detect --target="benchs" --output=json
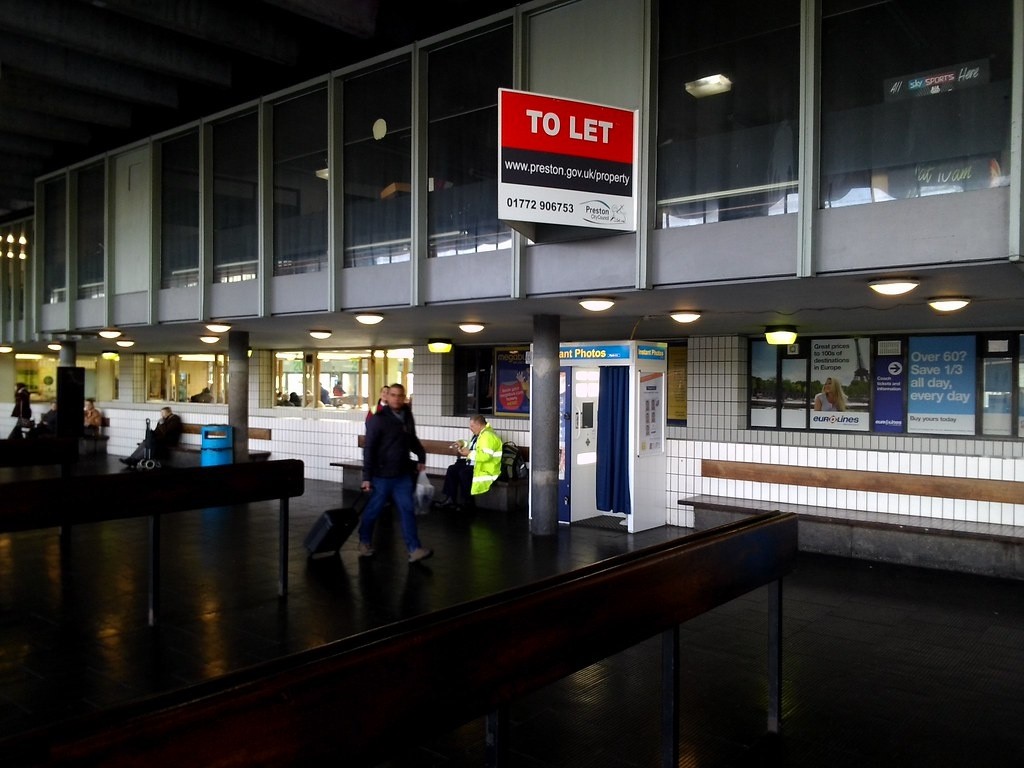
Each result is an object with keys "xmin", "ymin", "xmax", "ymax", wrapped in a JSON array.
[
  {"xmin": 138, "ymin": 423, "xmax": 272, "ymax": 469},
  {"xmin": 40, "ymin": 413, "xmax": 109, "ymax": 456},
  {"xmin": 676, "ymin": 459, "xmax": 1024, "ymax": 582},
  {"xmin": 330, "ymin": 436, "xmax": 528, "ymax": 513}
]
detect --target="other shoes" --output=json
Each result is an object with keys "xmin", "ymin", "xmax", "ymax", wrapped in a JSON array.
[
  {"xmin": 119, "ymin": 457, "xmax": 140, "ymax": 468},
  {"xmin": 437, "ymin": 497, "xmax": 456, "ymax": 507},
  {"xmin": 406, "ymin": 547, "xmax": 434, "ymax": 563},
  {"xmin": 356, "ymin": 540, "xmax": 376, "ymax": 557}
]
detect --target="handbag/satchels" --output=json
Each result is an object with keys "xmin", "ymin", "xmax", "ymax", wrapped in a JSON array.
[{"xmin": 416, "ymin": 469, "xmax": 434, "ymax": 508}]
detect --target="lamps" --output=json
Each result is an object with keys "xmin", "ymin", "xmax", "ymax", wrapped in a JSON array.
[
  {"xmin": 116, "ymin": 340, "xmax": 135, "ymax": 348},
  {"xmin": 310, "ymin": 330, "xmax": 332, "ymax": 339},
  {"xmin": 354, "ymin": 313, "xmax": 384, "ymax": 325},
  {"xmin": 669, "ymin": 311, "xmax": 702, "ymax": 324},
  {"xmin": 577, "ymin": 297, "xmax": 616, "ymax": 311},
  {"xmin": 764, "ymin": 325, "xmax": 799, "ymax": 344},
  {"xmin": 199, "ymin": 336, "xmax": 220, "ymax": 344},
  {"xmin": 684, "ymin": 72, "xmax": 732, "ymax": 100},
  {"xmin": 457, "ymin": 322, "xmax": 486, "ymax": 333},
  {"xmin": 102, "ymin": 350, "xmax": 118, "ymax": 360},
  {"xmin": 47, "ymin": 343, "xmax": 61, "ymax": 350},
  {"xmin": 926, "ymin": 298, "xmax": 971, "ymax": 312},
  {"xmin": 380, "ymin": 182, "xmax": 411, "ymax": 201},
  {"xmin": 867, "ymin": 279, "xmax": 920, "ymax": 295},
  {"xmin": 205, "ymin": 323, "xmax": 232, "ymax": 333},
  {"xmin": 98, "ymin": 330, "xmax": 122, "ymax": 339},
  {"xmin": 314, "ymin": 167, "xmax": 328, "ymax": 181},
  {"xmin": 0, "ymin": 346, "xmax": 13, "ymax": 354},
  {"xmin": 427, "ymin": 338, "xmax": 453, "ymax": 353}
]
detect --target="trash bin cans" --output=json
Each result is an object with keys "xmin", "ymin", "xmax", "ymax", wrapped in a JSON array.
[{"xmin": 200, "ymin": 425, "xmax": 233, "ymax": 466}]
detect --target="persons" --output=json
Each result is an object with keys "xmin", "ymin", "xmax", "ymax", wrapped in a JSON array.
[
  {"xmin": 289, "ymin": 392, "xmax": 301, "ymax": 407},
  {"xmin": 333, "ymin": 381, "xmax": 346, "ymax": 396},
  {"xmin": 305, "ymin": 389, "xmax": 313, "ymax": 405},
  {"xmin": 814, "ymin": 376, "xmax": 849, "ymax": 412},
  {"xmin": 179, "ymin": 379, "xmax": 187, "ymax": 402},
  {"xmin": 275, "ymin": 389, "xmax": 295, "ymax": 406},
  {"xmin": 365, "ymin": 385, "xmax": 390, "ymax": 427},
  {"xmin": 37, "ymin": 399, "xmax": 57, "ymax": 428},
  {"xmin": 118, "ymin": 407, "xmax": 183, "ymax": 467},
  {"xmin": 438, "ymin": 414, "xmax": 503, "ymax": 509},
  {"xmin": 333, "ymin": 398, "xmax": 345, "ymax": 409},
  {"xmin": 11, "ymin": 381, "xmax": 32, "ymax": 425},
  {"xmin": 356, "ymin": 383, "xmax": 434, "ymax": 562},
  {"xmin": 82, "ymin": 398, "xmax": 102, "ymax": 453},
  {"xmin": 320, "ymin": 382, "xmax": 330, "ymax": 404}
]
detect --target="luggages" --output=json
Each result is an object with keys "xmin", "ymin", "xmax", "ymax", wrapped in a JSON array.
[{"xmin": 303, "ymin": 484, "xmax": 374, "ymax": 557}]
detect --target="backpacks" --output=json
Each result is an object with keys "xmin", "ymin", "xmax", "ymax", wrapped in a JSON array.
[{"xmin": 499, "ymin": 441, "xmax": 529, "ymax": 482}]
